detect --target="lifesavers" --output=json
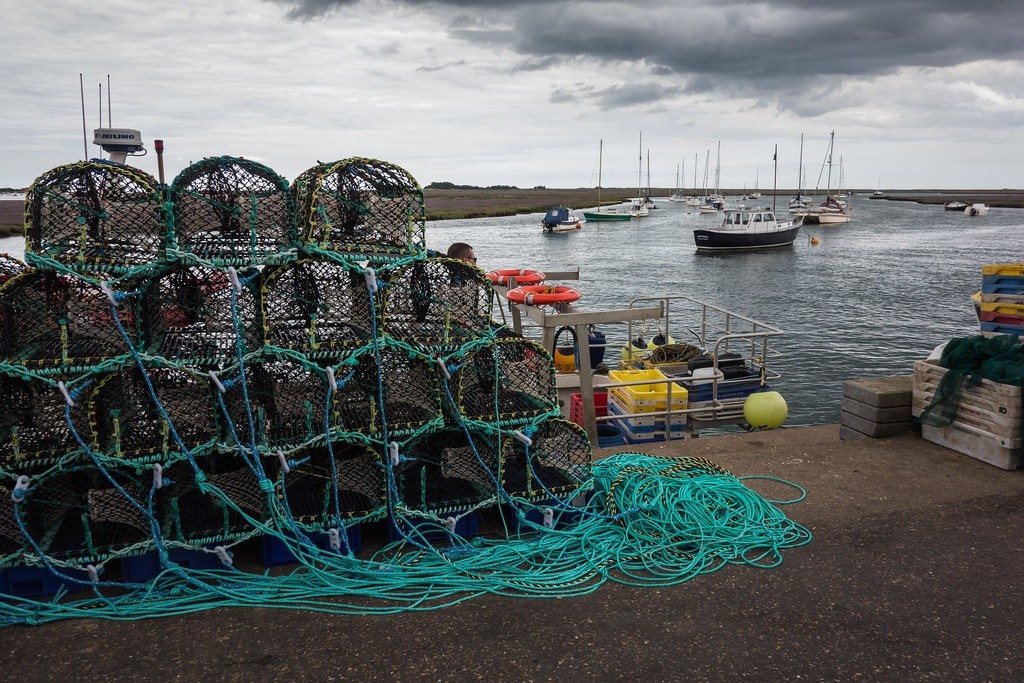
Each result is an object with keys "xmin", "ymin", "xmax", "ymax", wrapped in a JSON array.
[
  {"xmin": 506, "ymin": 285, "xmax": 581, "ymax": 305},
  {"xmin": 484, "ymin": 268, "xmax": 546, "ymax": 286}
]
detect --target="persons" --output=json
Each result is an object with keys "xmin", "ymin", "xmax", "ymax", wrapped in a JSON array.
[{"xmin": 447, "ymin": 242, "xmax": 477, "ymax": 268}]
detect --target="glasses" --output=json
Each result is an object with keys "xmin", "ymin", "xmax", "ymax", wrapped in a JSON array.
[{"xmin": 465, "ymin": 257, "xmax": 477, "ymax": 262}]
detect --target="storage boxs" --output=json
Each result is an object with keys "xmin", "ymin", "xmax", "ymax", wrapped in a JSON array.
[
  {"xmin": 912, "ymin": 262, "xmax": 1024, "ymax": 472},
  {"xmin": 569, "ymin": 351, "xmax": 766, "ymax": 445}
]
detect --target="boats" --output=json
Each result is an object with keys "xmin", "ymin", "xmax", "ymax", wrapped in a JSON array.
[
  {"xmin": 944, "ymin": 200, "xmax": 969, "ymax": 211},
  {"xmin": 874, "ymin": 190, "xmax": 882, "ymax": 195},
  {"xmin": 541, "ymin": 207, "xmax": 581, "ymax": 234},
  {"xmin": 693, "ymin": 207, "xmax": 804, "ymax": 249}
]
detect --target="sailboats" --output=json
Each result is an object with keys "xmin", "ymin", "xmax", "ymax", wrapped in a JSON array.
[
  {"xmin": 794, "ymin": 129, "xmax": 852, "ymax": 223},
  {"xmin": 669, "ymin": 140, "xmax": 725, "ymax": 213},
  {"xmin": 584, "ymin": 139, "xmax": 631, "ymax": 221},
  {"xmin": 788, "ymin": 130, "xmax": 813, "ymax": 212},
  {"xmin": 624, "ymin": 131, "xmax": 655, "ymax": 217},
  {"xmin": 742, "ymin": 182, "xmax": 747, "ymax": 200},
  {"xmin": 749, "ymin": 165, "xmax": 762, "ymax": 199}
]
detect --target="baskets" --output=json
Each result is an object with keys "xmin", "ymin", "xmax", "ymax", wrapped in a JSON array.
[
  {"xmin": 570, "ymin": 349, "xmax": 763, "ymax": 444},
  {"xmin": 971, "ymin": 263, "xmax": 1024, "ymax": 343}
]
